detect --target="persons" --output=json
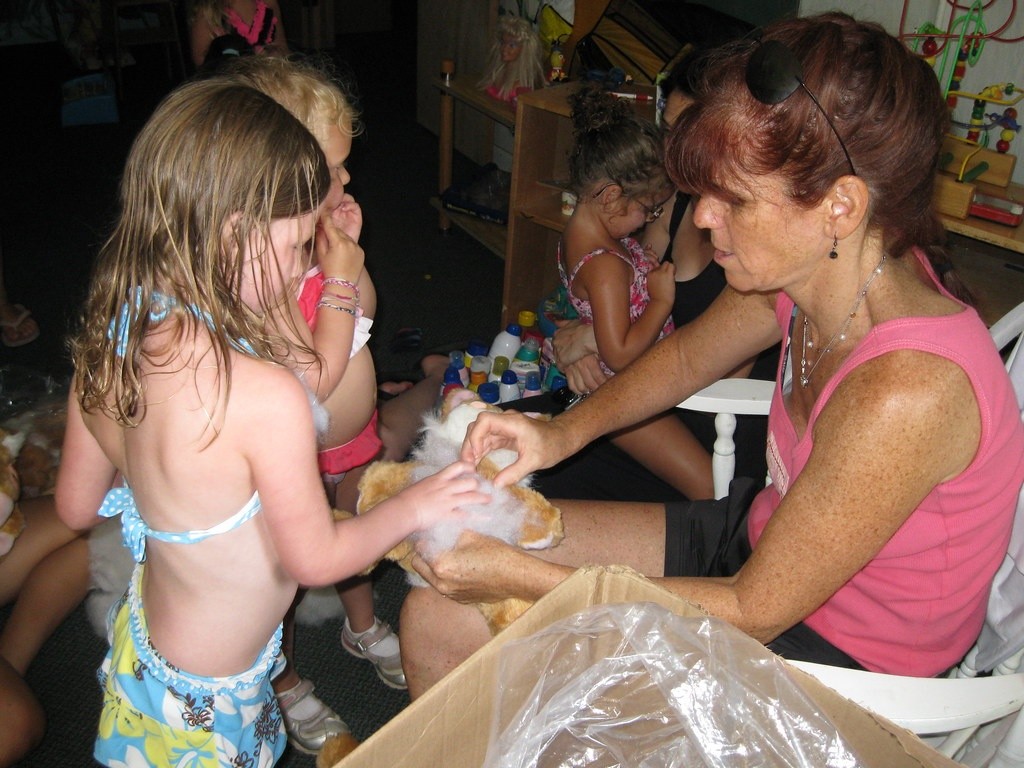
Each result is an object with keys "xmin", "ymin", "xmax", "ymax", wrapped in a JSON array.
[
  {"xmin": 397, "ymin": 10, "xmax": 1024, "ymax": 708},
  {"xmin": 0, "ymin": 0, "xmax": 413, "ymax": 767},
  {"xmin": 49, "ymin": 78, "xmax": 494, "ymax": 768}
]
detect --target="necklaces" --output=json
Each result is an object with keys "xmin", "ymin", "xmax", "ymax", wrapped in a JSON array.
[{"xmin": 799, "ymin": 256, "xmax": 886, "ymax": 389}]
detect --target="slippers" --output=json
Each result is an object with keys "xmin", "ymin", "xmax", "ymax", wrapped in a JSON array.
[{"xmin": 0, "ymin": 304, "xmax": 39, "ymax": 347}]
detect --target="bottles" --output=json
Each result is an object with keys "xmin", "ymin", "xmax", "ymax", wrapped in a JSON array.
[
  {"xmin": 432, "ymin": 35, "xmax": 468, "ymax": 81},
  {"xmin": 561, "ymin": 189, "xmax": 578, "ymax": 216},
  {"xmin": 436, "ymin": 309, "xmax": 581, "ymax": 418}
]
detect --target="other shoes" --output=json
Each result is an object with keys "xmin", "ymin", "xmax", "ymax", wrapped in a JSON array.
[
  {"xmin": 341, "ymin": 620, "xmax": 408, "ymax": 688},
  {"xmin": 276, "ymin": 678, "xmax": 350, "ymax": 756}
]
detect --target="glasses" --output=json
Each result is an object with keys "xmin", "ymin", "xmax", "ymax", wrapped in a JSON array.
[
  {"xmin": 593, "ymin": 182, "xmax": 664, "ymax": 220},
  {"xmin": 737, "ymin": 25, "xmax": 857, "ymax": 175}
]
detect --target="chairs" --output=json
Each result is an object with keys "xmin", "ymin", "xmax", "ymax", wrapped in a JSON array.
[{"xmin": 560, "ymin": 303, "xmax": 1024, "ymax": 768}]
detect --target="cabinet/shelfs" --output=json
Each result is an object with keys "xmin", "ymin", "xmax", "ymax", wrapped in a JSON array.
[
  {"xmin": 498, "ymin": 81, "xmax": 655, "ymax": 336},
  {"xmin": 426, "ymin": 60, "xmax": 517, "ymax": 260}
]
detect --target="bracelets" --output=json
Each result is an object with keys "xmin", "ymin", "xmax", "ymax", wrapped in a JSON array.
[
  {"xmin": 320, "ymin": 294, "xmax": 358, "ymax": 309},
  {"xmin": 317, "ymin": 302, "xmax": 355, "ymax": 317},
  {"xmin": 321, "ymin": 278, "xmax": 360, "ymax": 298}
]
